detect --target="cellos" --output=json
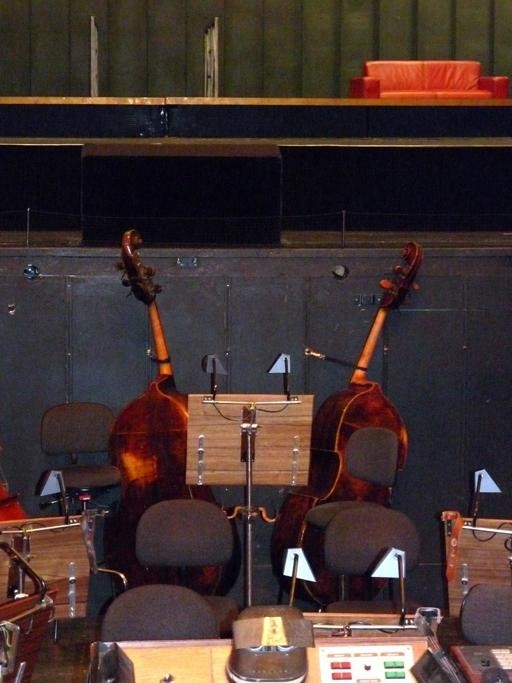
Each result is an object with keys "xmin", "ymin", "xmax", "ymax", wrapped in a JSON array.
[
  {"xmin": 271, "ymin": 240, "xmax": 423, "ymax": 606},
  {"xmin": 100, "ymin": 227, "xmax": 239, "ymax": 593}
]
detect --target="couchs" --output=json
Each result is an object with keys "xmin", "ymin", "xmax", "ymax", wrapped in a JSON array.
[{"xmin": 350, "ymin": 59, "xmax": 508, "ymax": 101}]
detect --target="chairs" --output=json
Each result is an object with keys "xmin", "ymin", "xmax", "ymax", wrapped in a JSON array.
[
  {"xmin": 129, "ymin": 496, "xmax": 240, "ymax": 639},
  {"xmin": 87, "ymin": 584, "xmax": 222, "ymax": 681},
  {"xmin": 323, "ymin": 503, "xmax": 424, "ymax": 616},
  {"xmin": 293, "ymin": 426, "xmax": 401, "ymax": 614},
  {"xmin": 39, "ymin": 401, "xmax": 129, "ymax": 645},
  {"xmin": 457, "ymin": 581, "xmax": 511, "ymax": 646}
]
